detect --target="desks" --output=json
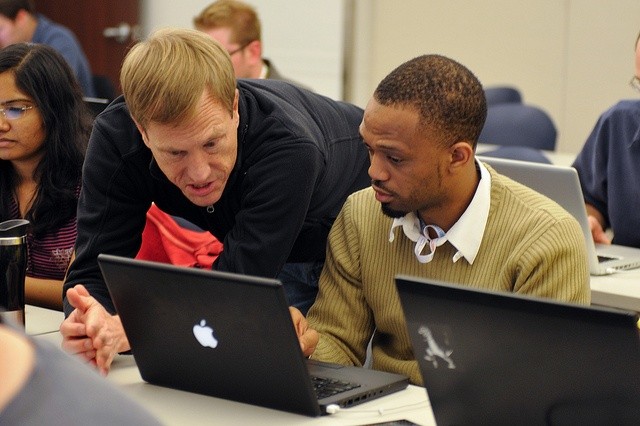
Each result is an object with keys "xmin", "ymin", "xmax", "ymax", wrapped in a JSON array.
[
  {"xmin": 591, "ymin": 242, "xmax": 640, "ymax": 315},
  {"xmin": 36, "ymin": 330, "xmax": 436, "ymax": 426},
  {"xmin": 24, "ymin": 303, "xmax": 71, "ymax": 334}
]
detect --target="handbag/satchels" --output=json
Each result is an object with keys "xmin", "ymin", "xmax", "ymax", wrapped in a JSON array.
[{"xmin": 135, "ymin": 202, "xmax": 224, "ymax": 270}]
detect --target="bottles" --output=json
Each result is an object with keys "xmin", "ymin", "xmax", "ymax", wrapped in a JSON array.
[{"xmin": 1, "ymin": 219, "xmax": 31, "ymax": 329}]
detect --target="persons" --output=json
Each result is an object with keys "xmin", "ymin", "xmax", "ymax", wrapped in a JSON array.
[
  {"xmin": 60, "ymin": 24, "xmax": 374, "ymax": 388},
  {"xmin": 193, "ymin": 0, "xmax": 302, "ymax": 84},
  {"xmin": 0, "ymin": 0, "xmax": 94, "ymax": 101},
  {"xmin": 570, "ymin": 29, "xmax": 639, "ymax": 249},
  {"xmin": 0, "ymin": 41, "xmax": 95, "ymax": 310},
  {"xmin": 285, "ymin": 52, "xmax": 591, "ymax": 387}
]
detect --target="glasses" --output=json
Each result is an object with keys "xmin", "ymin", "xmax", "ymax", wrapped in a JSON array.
[
  {"xmin": 0, "ymin": 104, "xmax": 37, "ymax": 120},
  {"xmin": 630, "ymin": 76, "xmax": 639, "ymax": 94},
  {"xmin": 229, "ymin": 42, "xmax": 251, "ymax": 55}
]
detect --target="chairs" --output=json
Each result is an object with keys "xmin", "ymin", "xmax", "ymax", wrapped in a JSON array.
[
  {"xmin": 484, "ymin": 87, "xmax": 521, "ymax": 105},
  {"xmin": 477, "ymin": 104, "xmax": 556, "ymax": 150}
]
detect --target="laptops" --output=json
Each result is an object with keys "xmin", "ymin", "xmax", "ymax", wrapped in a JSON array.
[
  {"xmin": 81, "ymin": 91, "xmax": 110, "ymax": 115},
  {"xmin": 392, "ymin": 273, "xmax": 634, "ymax": 425},
  {"xmin": 96, "ymin": 251, "xmax": 411, "ymax": 420},
  {"xmin": 477, "ymin": 154, "xmax": 640, "ymax": 279}
]
